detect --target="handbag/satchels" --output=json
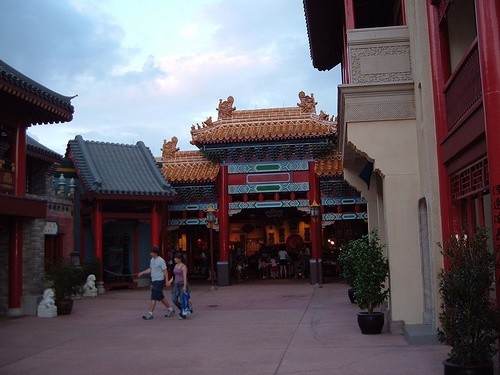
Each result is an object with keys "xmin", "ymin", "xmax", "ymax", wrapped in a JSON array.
[{"xmin": 180, "ymin": 291, "xmax": 193, "ymax": 316}]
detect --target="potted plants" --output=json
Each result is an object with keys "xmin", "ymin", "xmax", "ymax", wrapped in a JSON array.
[
  {"xmin": 434, "ymin": 221, "xmax": 500, "ymax": 375},
  {"xmin": 40, "ymin": 256, "xmax": 88, "ymax": 314},
  {"xmin": 337, "ymin": 225, "xmax": 390, "ymax": 334}
]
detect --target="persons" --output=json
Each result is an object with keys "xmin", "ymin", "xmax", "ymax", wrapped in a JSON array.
[
  {"xmin": 231, "ymin": 244, "xmax": 310, "ymax": 282},
  {"xmin": 167, "ymin": 253, "xmax": 189, "ymax": 320},
  {"xmin": 137, "ymin": 247, "xmax": 176, "ymax": 320}
]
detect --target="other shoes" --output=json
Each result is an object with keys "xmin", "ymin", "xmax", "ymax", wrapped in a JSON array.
[
  {"xmin": 177, "ymin": 314, "xmax": 185, "ymax": 320},
  {"xmin": 142, "ymin": 312, "xmax": 153, "ymax": 320},
  {"xmin": 164, "ymin": 309, "xmax": 175, "ymax": 318}
]
